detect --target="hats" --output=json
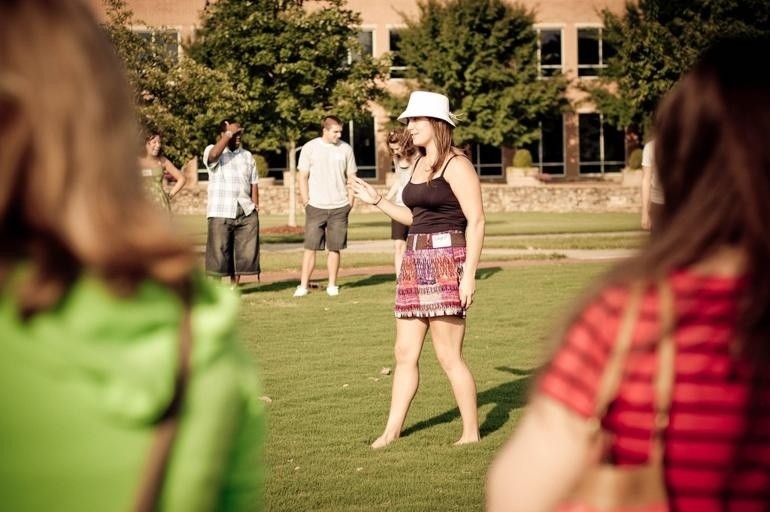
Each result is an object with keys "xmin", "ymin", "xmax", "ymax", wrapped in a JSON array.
[{"xmin": 403, "ymin": 91, "xmax": 455, "ymax": 124}]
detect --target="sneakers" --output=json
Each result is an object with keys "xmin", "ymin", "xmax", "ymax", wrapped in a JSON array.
[
  {"xmin": 326, "ymin": 285, "xmax": 339, "ymax": 297},
  {"xmin": 293, "ymin": 286, "xmax": 308, "ymax": 296}
]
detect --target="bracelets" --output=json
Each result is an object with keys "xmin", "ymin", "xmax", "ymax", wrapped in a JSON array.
[
  {"xmin": 374, "ymin": 196, "xmax": 384, "ymax": 205},
  {"xmin": 303, "ymin": 199, "xmax": 309, "ymax": 207}
]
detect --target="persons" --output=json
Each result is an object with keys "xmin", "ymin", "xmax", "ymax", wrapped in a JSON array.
[
  {"xmin": 483, "ymin": 36, "xmax": 770, "ymax": 511},
  {"xmin": 0, "ymin": 0, "xmax": 268, "ymax": 511},
  {"xmin": 348, "ymin": 90, "xmax": 485, "ymax": 449},
  {"xmin": 641, "ymin": 139, "xmax": 671, "ymax": 242},
  {"xmin": 202, "ymin": 119, "xmax": 261, "ymax": 289},
  {"xmin": 386, "ymin": 126, "xmax": 420, "ymax": 286},
  {"xmin": 294, "ymin": 115, "xmax": 358, "ymax": 297},
  {"xmin": 135, "ymin": 126, "xmax": 186, "ymax": 210}
]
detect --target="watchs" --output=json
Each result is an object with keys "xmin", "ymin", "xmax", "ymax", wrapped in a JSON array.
[
  {"xmin": 167, "ymin": 193, "xmax": 172, "ymax": 199},
  {"xmin": 225, "ymin": 129, "xmax": 233, "ymax": 138}
]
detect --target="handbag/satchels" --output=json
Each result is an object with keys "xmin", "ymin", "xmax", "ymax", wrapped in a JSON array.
[{"xmin": 548, "ymin": 266, "xmax": 673, "ymax": 512}]
